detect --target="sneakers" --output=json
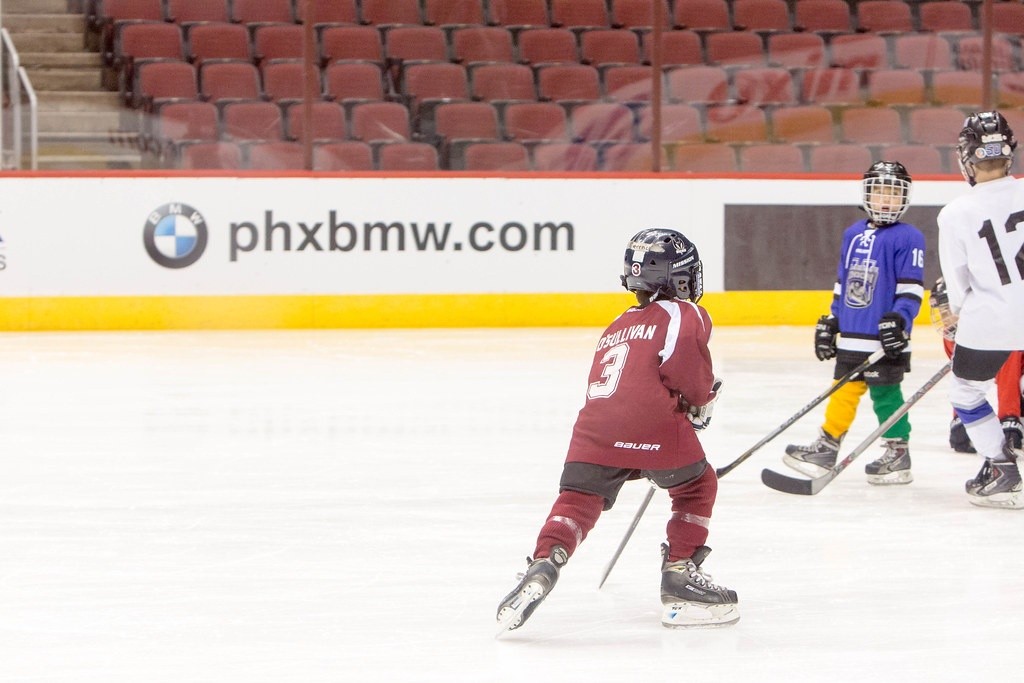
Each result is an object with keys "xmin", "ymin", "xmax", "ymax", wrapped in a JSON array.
[
  {"xmin": 783, "ymin": 427, "xmax": 848, "ymax": 480},
  {"xmin": 865, "ymin": 440, "xmax": 914, "ymax": 485},
  {"xmin": 493, "ymin": 544, "xmax": 567, "ymax": 639},
  {"xmin": 1001, "ymin": 416, "xmax": 1023, "ymax": 458},
  {"xmin": 965, "ymin": 457, "xmax": 1023, "ymax": 509},
  {"xmin": 949, "ymin": 415, "xmax": 977, "ymax": 454},
  {"xmin": 659, "ymin": 543, "xmax": 740, "ymax": 626}
]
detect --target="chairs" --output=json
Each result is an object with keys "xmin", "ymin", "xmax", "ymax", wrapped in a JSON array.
[{"xmin": 83, "ymin": 0, "xmax": 1024, "ymax": 172}]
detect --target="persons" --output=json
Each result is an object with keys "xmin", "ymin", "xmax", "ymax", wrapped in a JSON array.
[
  {"xmin": 497, "ymin": 229, "xmax": 740, "ymax": 632},
  {"xmin": 937, "ymin": 111, "xmax": 1024, "ymax": 507},
  {"xmin": 784, "ymin": 160, "xmax": 925, "ymax": 484},
  {"xmin": 929, "ymin": 279, "xmax": 1024, "ymax": 459}
]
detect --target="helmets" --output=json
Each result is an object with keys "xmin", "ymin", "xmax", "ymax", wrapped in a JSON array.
[
  {"xmin": 956, "ymin": 111, "xmax": 1018, "ymax": 187},
  {"xmin": 620, "ymin": 228, "xmax": 704, "ymax": 303},
  {"xmin": 930, "ymin": 277, "xmax": 959, "ymax": 342},
  {"xmin": 858, "ymin": 161, "xmax": 912, "ymax": 225}
]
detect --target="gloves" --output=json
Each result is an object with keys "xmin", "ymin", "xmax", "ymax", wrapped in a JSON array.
[
  {"xmin": 687, "ymin": 377, "xmax": 724, "ymax": 430},
  {"xmin": 814, "ymin": 315, "xmax": 839, "ymax": 361},
  {"xmin": 879, "ymin": 312, "xmax": 909, "ymax": 360}
]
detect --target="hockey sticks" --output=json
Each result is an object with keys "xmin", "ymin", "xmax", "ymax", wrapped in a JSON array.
[
  {"xmin": 597, "ymin": 404, "xmax": 700, "ymax": 590},
  {"xmin": 712, "ymin": 331, "xmax": 912, "ymax": 478},
  {"xmin": 761, "ymin": 358, "xmax": 955, "ymax": 496}
]
